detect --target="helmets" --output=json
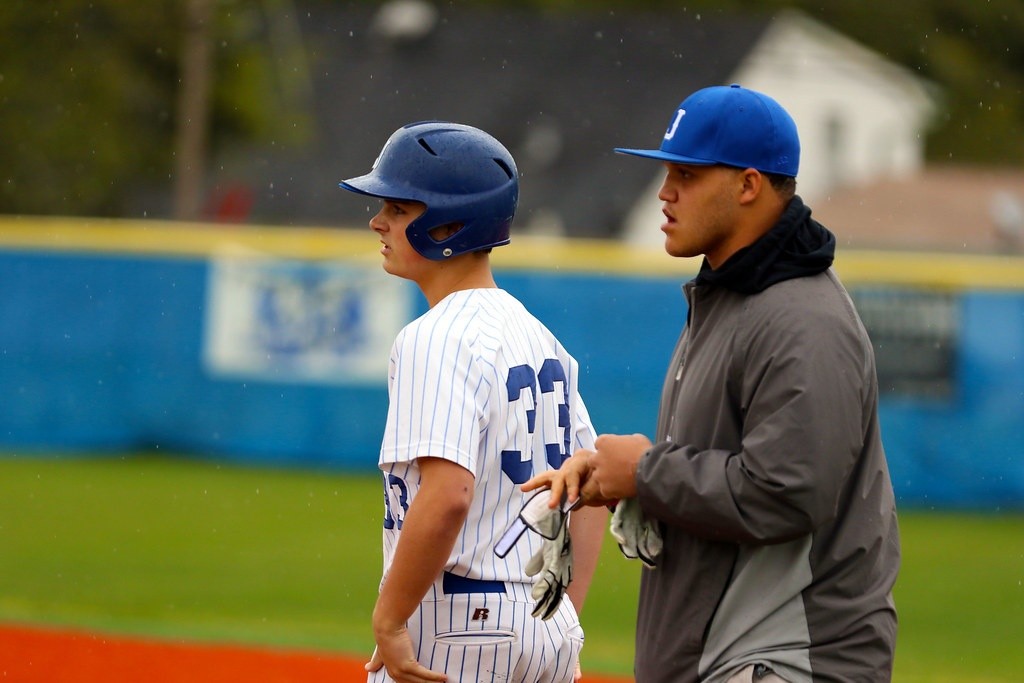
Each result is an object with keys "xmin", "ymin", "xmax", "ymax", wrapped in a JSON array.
[{"xmin": 339, "ymin": 119, "xmax": 518, "ymax": 259}]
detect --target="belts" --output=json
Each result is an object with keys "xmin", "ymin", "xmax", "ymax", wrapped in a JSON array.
[{"xmin": 444, "ymin": 571, "xmax": 506, "ymax": 594}]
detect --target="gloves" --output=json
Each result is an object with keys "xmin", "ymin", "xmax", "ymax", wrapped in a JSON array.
[
  {"xmin": 526, "ymin": 516, "xmax": 573, "ymax": 622},
  {"xmin": 610, "ymin": 497, "xmax": 664, "ymax": 568}
]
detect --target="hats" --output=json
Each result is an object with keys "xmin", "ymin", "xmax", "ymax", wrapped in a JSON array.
[{"xmin": 614, "ymin": 84, "xmax": 800, "ymax": 176}]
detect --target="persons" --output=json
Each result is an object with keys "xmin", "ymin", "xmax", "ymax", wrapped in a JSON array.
[
  {"xmin": 512, "ymin": 85, "xmax": 904, "ymax": 683},
  {"xmin": 334, "ymin": 120, "xmax": 615, "ymax": 683}
]
